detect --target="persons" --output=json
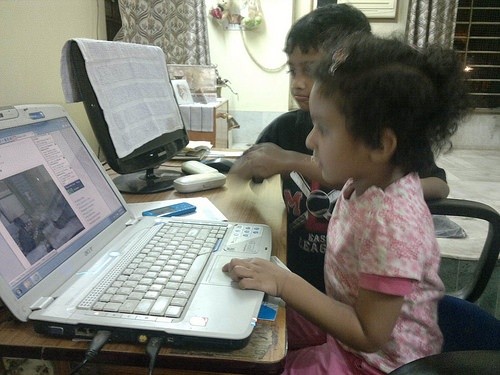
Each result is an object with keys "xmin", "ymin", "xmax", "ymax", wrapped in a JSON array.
[
  {"xmin": 226, "ymin": 4, "xmax": 450, "ymax": 290},
  {"xmin": 223, "ymin": 38, "xmax": 474, "ymax": 375}
]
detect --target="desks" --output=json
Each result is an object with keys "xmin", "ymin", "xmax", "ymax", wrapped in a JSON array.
[{"xmin": 0, "ymin": 148, "xmax": 287, "ymax": 375}]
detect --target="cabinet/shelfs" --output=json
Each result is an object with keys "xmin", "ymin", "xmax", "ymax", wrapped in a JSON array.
[{"xmin": 179, "ymin": 99, "xmax": 229, "ymax": 148}]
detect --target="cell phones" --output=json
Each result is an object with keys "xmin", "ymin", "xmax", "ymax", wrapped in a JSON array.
[{"xmin": 142, "ymin": 200, "xmax": 193, "ymax": 218}]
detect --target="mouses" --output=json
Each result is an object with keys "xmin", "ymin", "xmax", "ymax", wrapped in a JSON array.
[{"xmin": 200, "ymin": 157, "xmax": 235, "ymax": 171}]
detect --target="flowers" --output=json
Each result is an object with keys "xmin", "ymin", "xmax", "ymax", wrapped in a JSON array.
[{"xmin": 209, "ymin": 0, "xmax": 263, "ymax": 31}]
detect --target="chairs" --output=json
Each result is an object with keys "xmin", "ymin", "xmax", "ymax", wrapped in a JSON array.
[{"xmin": 387, "ymin": 198, "xmax": 500, "ymax": 375}]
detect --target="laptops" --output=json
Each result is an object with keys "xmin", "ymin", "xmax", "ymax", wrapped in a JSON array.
[{"xmin": 0, "ymin": 103, "xmax": 275, "ymax": 350}]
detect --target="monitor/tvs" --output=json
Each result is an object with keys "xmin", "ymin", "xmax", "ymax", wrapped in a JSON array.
[{"xmin": 69, "ymin": 41, "xmax": 189, "ymax": 194}]
